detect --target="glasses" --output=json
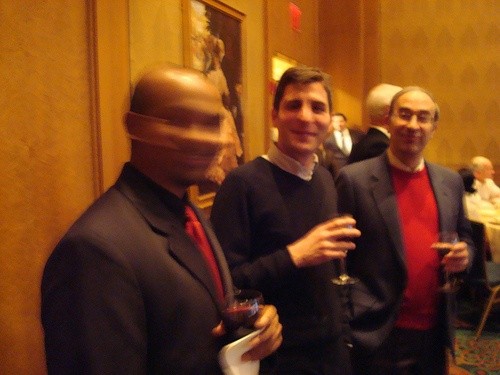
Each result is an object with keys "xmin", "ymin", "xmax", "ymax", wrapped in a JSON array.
[{"xmin": 391, "ymin": 111, "xmax": 436, "ymax": 123}]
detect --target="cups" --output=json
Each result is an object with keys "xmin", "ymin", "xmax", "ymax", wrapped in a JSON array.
[{"xmin": 218, "ymin": 289, "xmax": 264, "ymax": 342}]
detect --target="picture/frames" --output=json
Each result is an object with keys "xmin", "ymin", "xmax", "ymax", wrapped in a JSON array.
[{"xmin": 180, "ymin": 0, "xmax": 249, "ymax": 209}]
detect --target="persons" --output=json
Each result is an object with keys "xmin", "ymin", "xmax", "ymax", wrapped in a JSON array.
[
  {"xmin": 334, "ymin": 86, "xmax": 475, "ymax": 375},
  {"xmin": 210, "ymin": 65, "xmax": 361, "ymax": 375},
  {"xmin": 471, "ymin": 156, "xmax": 500, "ymax": 207},
  {"xmin": 457, "ymin": 168, "xmax": 492, "ymax": 209},
  {"xmin": 349, "ymin": 83, "xmax": 403, "ymax": 163},
  {"xmin": 324, "ymin": 113, "xmax": 365, "ymax": 170},
  {"xmin": 39, "ymin": 67, "xmax": 282, "ymax": 375}
]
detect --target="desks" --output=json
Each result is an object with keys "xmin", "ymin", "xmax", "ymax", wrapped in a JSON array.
[{"xmin": 466, "ymin": 196, "xmax": 500, "ymax": 263}]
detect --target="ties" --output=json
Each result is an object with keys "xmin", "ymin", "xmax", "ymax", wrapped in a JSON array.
[
  {"xmin": 341, "ymin": 131, "xmax": 348, "ymax": 157},
  {"xmin": 183, "ymin": 203, "xmax": 226, "ymax": 306}
]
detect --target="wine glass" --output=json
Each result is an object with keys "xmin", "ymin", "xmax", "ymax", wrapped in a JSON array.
[
  {"xmin": 326, "ymin": 214, "xmax": 358, "ymax": 285},
  {"xmin": 430, "ymin": 230, "xmax": 463, "ymax": 293}
]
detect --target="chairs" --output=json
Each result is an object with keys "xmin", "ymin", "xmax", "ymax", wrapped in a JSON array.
[{"xmin": 466, "ymin": 218, "xmax": 500, "ymax": 340}]
